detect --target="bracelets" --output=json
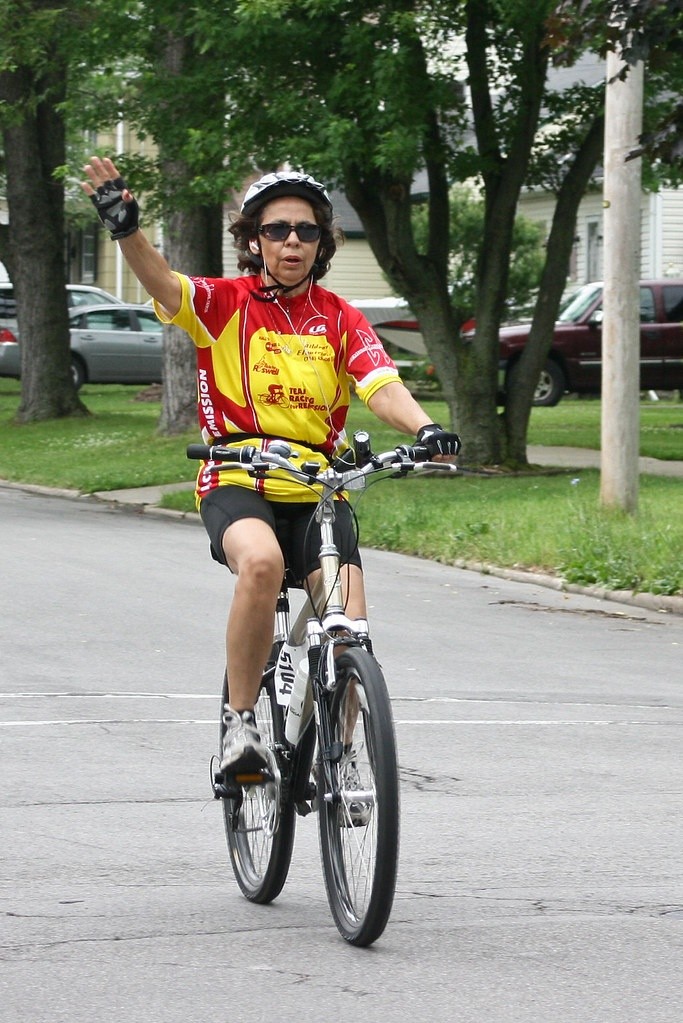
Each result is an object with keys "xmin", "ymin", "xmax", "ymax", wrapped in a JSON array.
[{"xmin": 110, "ymin": 225, "xmax": 138, "ymax": 241}]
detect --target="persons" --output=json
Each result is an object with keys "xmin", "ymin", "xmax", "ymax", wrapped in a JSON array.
[{"xmin": 76, "ymin": 152, "xmax": 463, "ymax": 830}]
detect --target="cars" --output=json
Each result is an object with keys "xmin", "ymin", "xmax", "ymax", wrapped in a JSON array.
[
  {"xmin": 0, "ymin": 303, "xmax": 172, "ymax": 392},
  {"xmin": 0, "ymin": 283, "xmax": 123, "ymax": 313}
]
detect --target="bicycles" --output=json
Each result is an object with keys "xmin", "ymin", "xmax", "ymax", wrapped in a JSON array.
[{"xmin": 183, "ymin": 430, "xmax": 463, "ymax": 949}]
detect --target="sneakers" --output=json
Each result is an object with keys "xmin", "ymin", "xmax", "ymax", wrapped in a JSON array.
[
  {"xmin": 311, "ymin": 756, "xmax": 372, "ymax": 828},
  {"xmin": 220, "ymin": 722, "xmax": 268, "ymax": 776}
]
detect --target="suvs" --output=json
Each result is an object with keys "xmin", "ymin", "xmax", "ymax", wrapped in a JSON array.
[{"xmin": 460, "ymin": 279, "xmax": 683, "ymax": 408}]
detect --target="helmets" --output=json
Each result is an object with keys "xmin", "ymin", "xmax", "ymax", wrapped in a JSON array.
[{"xmin": 239, "ymin": 172, "xmax": 334, "ymax": 225}]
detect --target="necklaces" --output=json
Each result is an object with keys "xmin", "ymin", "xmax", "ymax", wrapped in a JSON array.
[{"xmin": 259, "ymin": 271, "xmax": 313, "ymax": 354}]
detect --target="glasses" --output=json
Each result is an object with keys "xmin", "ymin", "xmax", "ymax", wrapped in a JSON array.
[{"xmin": 254, "ymin": 223, "xmax": 324, "ymax": 243}]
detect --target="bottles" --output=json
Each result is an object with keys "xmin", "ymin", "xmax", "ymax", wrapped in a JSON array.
[{"xmin": 285, "ymin": 657, "xmax": 309, "ymax": 746}]
